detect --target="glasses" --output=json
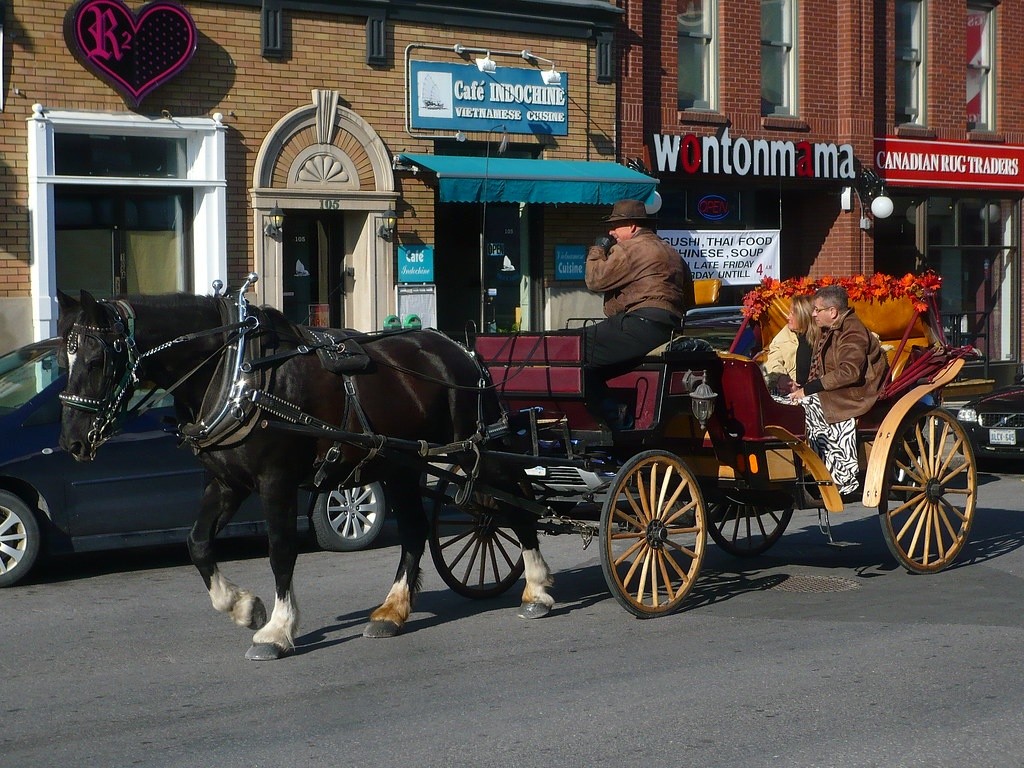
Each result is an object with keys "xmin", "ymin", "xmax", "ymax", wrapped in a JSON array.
[{"xmin": 813, "ymin": 306, "xmax": 835, "ymax": 314}]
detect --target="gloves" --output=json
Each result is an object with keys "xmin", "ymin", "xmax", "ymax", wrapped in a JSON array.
[{"xmin": 595, "ymin": 235, "xmax": 617, "ymax": 255}]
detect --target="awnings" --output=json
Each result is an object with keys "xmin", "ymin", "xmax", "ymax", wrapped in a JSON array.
[{"xmin": 400, "ymin": 154, "xmax": 658, "ymax": 205}]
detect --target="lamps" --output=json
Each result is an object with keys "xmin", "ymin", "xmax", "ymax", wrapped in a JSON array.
[
  {"xmin": 264, "ymin": 200, "xmax": 292, "ymax": 239},
  {"xmin": 859, "ymin": 168, "xmax": 893, "ymax": 219},
  {"xmin": 454, "ymin": 44, "xmax": 496, "ymax": 74},
  {"xmin": 520, "ymin": 49, "xmax": 561, "ymax": 86},
  {"xmin": 379, "ymin": 202, "xmax": 397, "ymax": 238},
  {"xmin": 644, "ymin": 190, "xmax": 662, "ymax": 214}
]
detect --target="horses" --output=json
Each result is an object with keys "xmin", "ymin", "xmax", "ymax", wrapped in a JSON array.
[{"xmin": 55, "ymin": 287, "xmax": 555, "ymax": 662}]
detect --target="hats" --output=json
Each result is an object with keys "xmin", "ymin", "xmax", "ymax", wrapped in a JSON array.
[{"xmin": 601, "ymin": 200, "xmax": 654, "ymax": 222}]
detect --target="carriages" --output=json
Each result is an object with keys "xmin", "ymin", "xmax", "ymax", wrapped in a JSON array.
[{"xmin": 58, "ymin": 271, "xmax": 996, "ymax": 662}]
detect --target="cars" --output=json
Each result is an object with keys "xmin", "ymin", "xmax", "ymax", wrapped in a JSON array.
[
  {"xmin": 521, "ymin": 455, "xmax": 722, "ymax": 527},
  {"xmin": 954, "ymin": 373, "xmax": 1024, "ymax": 471},
  {"xmin": 1, "ymin": 335, "xmax": 432, "ymax": 589}
]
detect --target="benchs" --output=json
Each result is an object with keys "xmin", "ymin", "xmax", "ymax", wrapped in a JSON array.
[
  {"xmin": 729, "ymin": 269, "xmax": 950, "ymax": 401},
  {"xmin": 593, "ymin": 334, "xmax": 723, "ymax": 428}
]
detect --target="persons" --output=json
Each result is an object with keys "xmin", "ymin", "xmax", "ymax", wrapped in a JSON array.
[
  {"xmin": 559, "ymin": 200, "xmax": 690, "ymax": 432},
  {"xmin": 763, "ymin": 286, "xmax": 889, "ymax": 501}
]
